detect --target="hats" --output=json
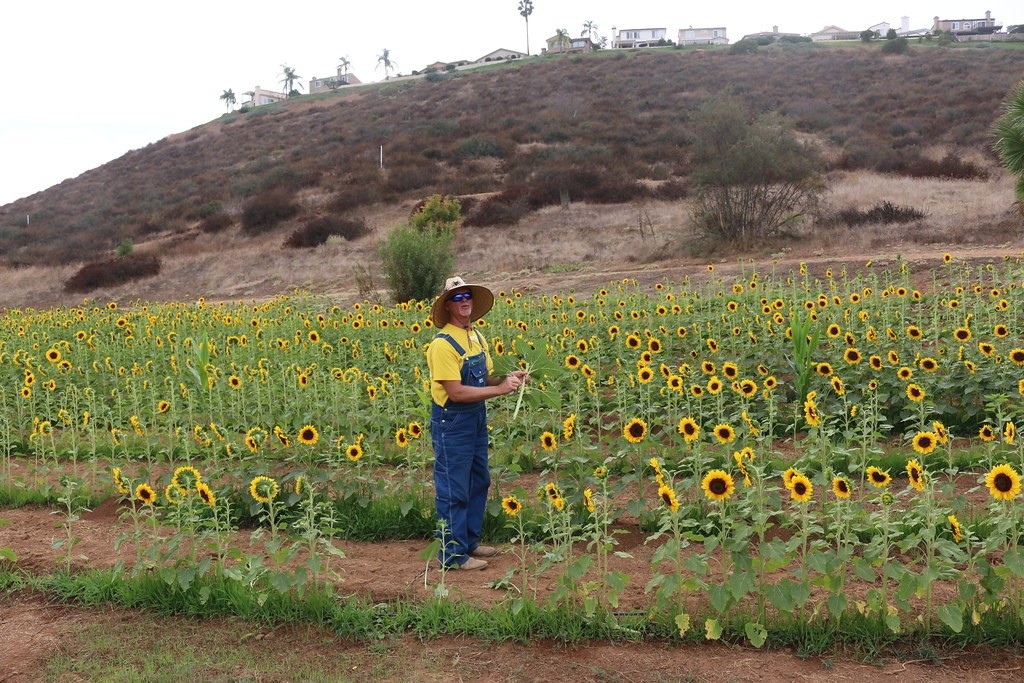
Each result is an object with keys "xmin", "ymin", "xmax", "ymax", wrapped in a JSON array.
[{"xmin": 431, "ymin": 277, "xmax": 496, "ymax": 329}]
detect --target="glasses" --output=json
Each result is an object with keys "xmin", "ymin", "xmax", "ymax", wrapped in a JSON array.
[{"xmin": 445, "ymin": 293, "xmax": 472, "ymax": 302}]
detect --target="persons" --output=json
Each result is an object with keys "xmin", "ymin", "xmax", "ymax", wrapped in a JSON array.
[{"xmin": 427, "ymin": 276, "xmax": 530, "ymax": 571}]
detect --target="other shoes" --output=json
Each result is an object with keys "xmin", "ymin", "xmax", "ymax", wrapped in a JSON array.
[
  {"xmin": 472, "ymin": 545, "xmax": 496, "ymax": 556},
  {"xmin": 441, "ymin": 557, "xmax": 489, "ymax": 571}
]
